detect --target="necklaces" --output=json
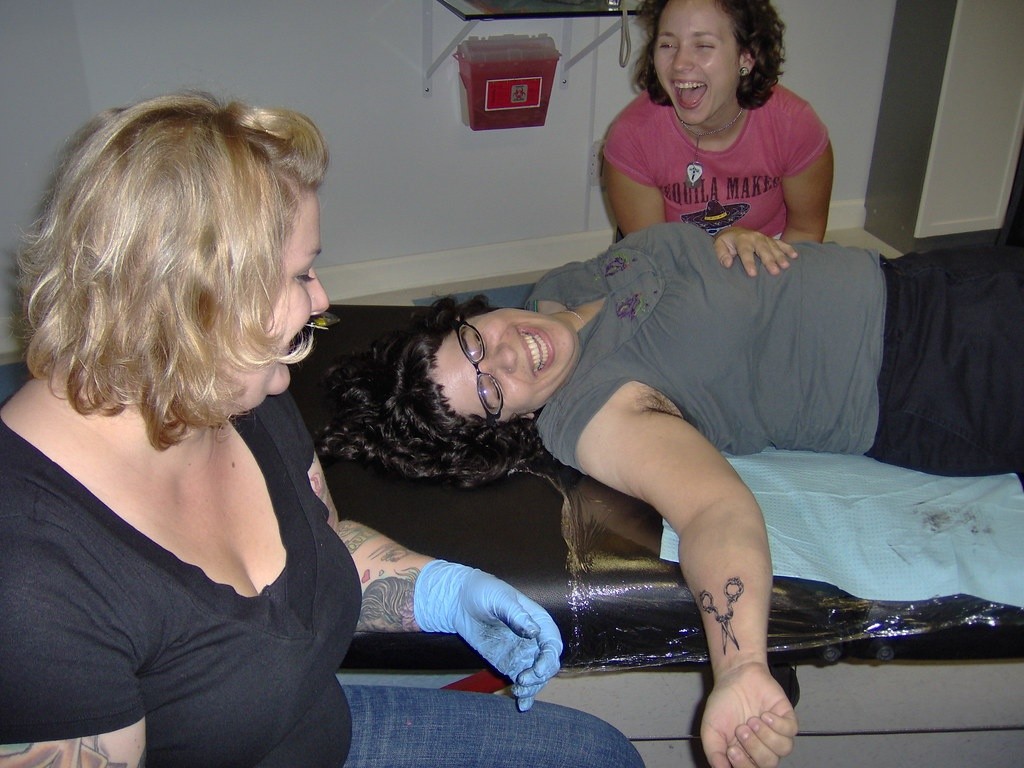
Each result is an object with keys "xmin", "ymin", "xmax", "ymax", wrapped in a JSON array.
[
  {"xmin": 681, "ymin": 109, "xmax": 744, "ymax": 189},
  {"xmin": 562, "ymin": 310, "xmax": 585, "ymax": 325}
]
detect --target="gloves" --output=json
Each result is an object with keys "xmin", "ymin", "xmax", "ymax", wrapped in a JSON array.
[{"xmin": 412, "ymin": 559, "xmax": 564, "ymax": 713}]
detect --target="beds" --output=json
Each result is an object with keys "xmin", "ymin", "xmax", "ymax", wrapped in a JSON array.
[{"xmin": 288, "ymin": 305, "xmax": 1024, "ymax": 767}]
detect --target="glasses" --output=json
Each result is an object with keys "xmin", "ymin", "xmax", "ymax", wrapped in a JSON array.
[{"xmin": 452, "ymin": 320, "xmax": 504, "ymax": 427}]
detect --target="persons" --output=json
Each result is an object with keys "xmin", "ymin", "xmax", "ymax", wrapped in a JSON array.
[
  {"xmin": 602, "ymin": 0, "xmax": 833, "ymax": 245},
  {"xmin": 320, "ymin": 221, "xmax": 1024, "ymax": 768},
  {"xmin": 0, "ymin": 94, "xmax": 647, "ymax": 768}
]
